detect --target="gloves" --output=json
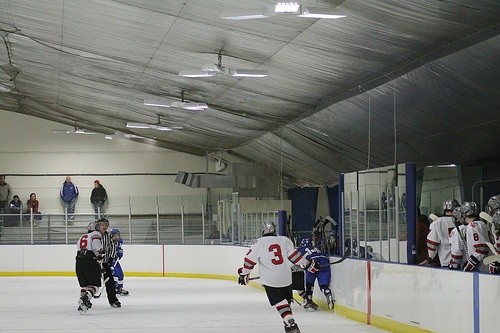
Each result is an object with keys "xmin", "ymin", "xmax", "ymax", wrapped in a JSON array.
[
  {"xmin": 118, "ymin": 249, "xmax": 123, "ymax": 259},
  {"xmin": 110, "ymin": 267, "xmax": 114, "ymax": 275},
  {"xmin": 424, "ymin": 256, "xmax": 499, "ymax": 274},
  {"xmin": 302, "ymin": 259, "xmax": 320, "ymax": 273},
  {"xmin": 237, "ymin": 268, "xmax": 250, "ymax": 285}
]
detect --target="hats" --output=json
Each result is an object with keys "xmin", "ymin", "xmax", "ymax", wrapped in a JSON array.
[{"xmin": 93, "ymin": 180, "xmax": 99, "ymax": 183}]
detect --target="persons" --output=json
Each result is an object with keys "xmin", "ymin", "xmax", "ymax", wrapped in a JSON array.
[
  {"xmin": 381, "ymin": 191, "xmax": 388, "ymax": 221},
  {"xmin": 298, "ymin": 231, "xmax": 335, "ymax": 309},
  {"xmin": 74, "ymin": 216, "xmax": 110, "ymax": 312},
  {"xmin": 60, "ymin": 176, "xmax": 79, "ymax": 224},
  {"xmin": 25, "ymin": 193, "xmax": 43, "ymax": 223},
  {"xmin": 8, "ymin": 194, "xmax": 26, "ymax": 224},
  {"xmin": 459, "ymin": 201, "xmax": 495, "ymax": 273},
  {"xmin": 447, "ymin": 207, "xmax": 473, "ymax": 271},
  {"xmin": 344, "ymin": 237, "xmax": 368, "ymax": 258},
  {"xmin": 239, "ymin": 220, "xmax": 320, "ymax": 333},
  {"xmin": 418, "ymin": 198, "xmax": 463, "ymax": 267},
  {"xmin": 322, "ymin": 233, "xmax": 341, "ymax": 255},
  {"xmin": 106, "ymin": 228, "xmax": 129, "ymax": 295},
  {"xmin": 0, "ymin": 174, "xmax": 12, "ymax": 225},
  {"xmin": 90, "ymin": 179, "xmax": 109, "ymax": 222},
  {"xmin": 85, "ymin": 221, "xmax": 121, "ymax": 308},
  {"xmin": 486, "ymin": 196, "xmax": 500, "ymax": 275}
]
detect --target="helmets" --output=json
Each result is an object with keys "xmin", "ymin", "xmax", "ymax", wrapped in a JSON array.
[
  {"xmin": 442, "ymin": 194, "xmax": 500, "ymax": 225},
  {"xmin": 87, "ymin": 218, "xmax": 121, "ymax": 241},
  {"xmin": 300, "ymin": 237, "xmax": 312, "ymax": 248},
  {"xmin": 364, "ymin": 246, "xmax": 373, "ymax": 251},
  {"xmin": 260, "ymin": 221, "xmax": 277, "ymax": 235},
  {"xmin": 344, "ymin": 237, "xmax": 358, "ymax": 247}
]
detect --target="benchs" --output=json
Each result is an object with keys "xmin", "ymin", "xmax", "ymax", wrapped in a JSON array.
[{"xmin": 1, "ymin": 211, "xmax": 230, "ymax": 245}]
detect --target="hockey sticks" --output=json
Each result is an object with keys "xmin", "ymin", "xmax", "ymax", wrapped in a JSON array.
[
  {"xmin": 93, "ymin": 256, "xmax": 120, "ymax": 299},
  {"xmin": 427, "ymin": 210, "xmax": 500, "ymax": 275},
  {"xmin": 248, "ymin": 249, "xmax": 350, "ymax": 281}
]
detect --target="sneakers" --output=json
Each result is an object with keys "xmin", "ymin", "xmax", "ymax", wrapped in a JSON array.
[
  {"xmin": 284, "ymin": 323, "xmax": 300, "ymax": 333},
  {"xmin": 77, "ymin": 288, "xmax": 129, "ymax": 314},
  {"xmin": 304, "ymin": 294, "xmax": 334, "ymax": 311}
]
